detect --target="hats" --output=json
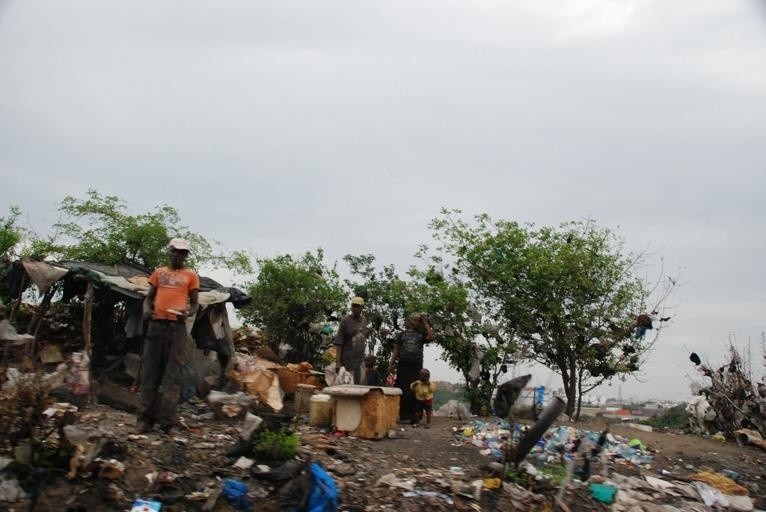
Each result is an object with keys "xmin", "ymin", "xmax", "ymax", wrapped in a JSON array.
[
  {"xmin": 351, "ymin": 297, "xmax": 364, "ymax": 306},
  {"xmin": 169, "ymin": 238, "xmax": 190, "ymax": 252},
  {"xmin": 197, "ymin": 290, "xmax": 231, "ymax": 305}
]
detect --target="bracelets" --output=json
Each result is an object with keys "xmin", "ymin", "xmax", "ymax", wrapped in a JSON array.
[{"xmin": 185, "ymin": 310, "xmax": 190, "ymax": 316}]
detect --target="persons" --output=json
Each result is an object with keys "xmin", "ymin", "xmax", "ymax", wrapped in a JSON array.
[
  {"xmin": 131, "ymin": 237, "xmax": 200, "ymax": 434},
  {"xmin": 411, "ymin": 369, "xmax": 436, "ymax": 428},
  {"xmin": 386, "ymin": 311, "xmax": 435, "ymax": 424},
  {"xmin": 333, "ymin": 296, "xmax": 367, "ymax": 389},
  {"xmin": 359, "ymin": 356, "xmax": 381, "ymax": 386}
]
